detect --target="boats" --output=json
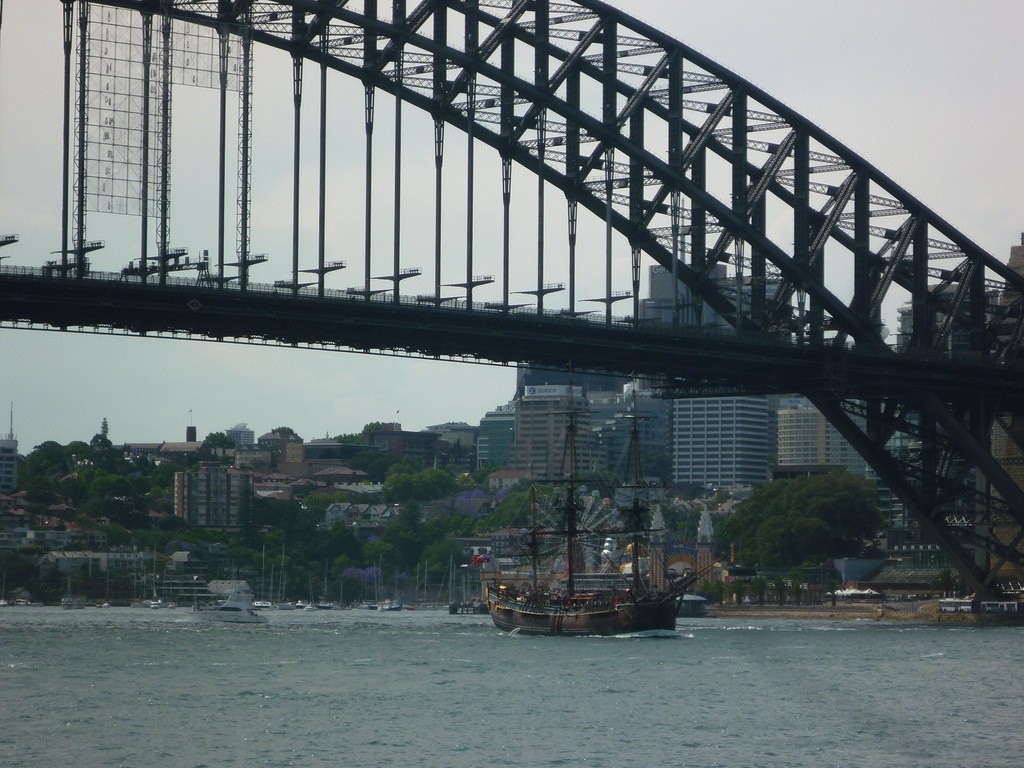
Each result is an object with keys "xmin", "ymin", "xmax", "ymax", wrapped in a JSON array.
[
  {"xmin": 468, "ymin": 377, "xmax": 754, "ymax": 632},
  {"xmin": 187, "ymin": 588, "xmax": 266, "ymax": 623},
  {"xmin": 1, "ymin": 597, "xmax": 416, "ymax": 612}
]
